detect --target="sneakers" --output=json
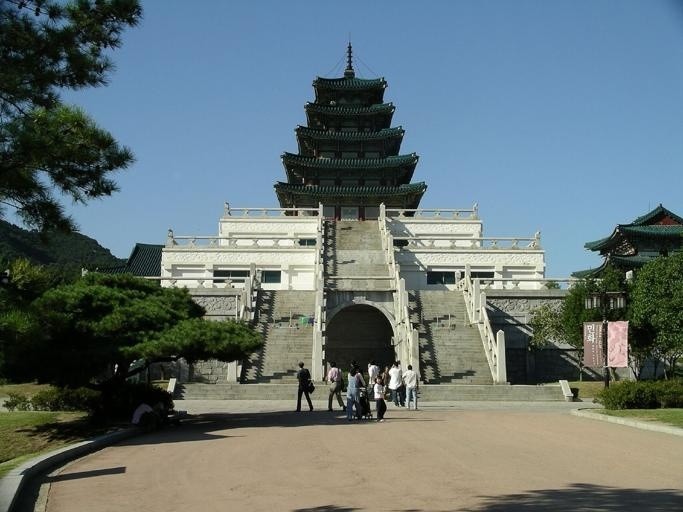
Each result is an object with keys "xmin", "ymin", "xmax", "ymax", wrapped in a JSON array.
[
  {"xmin": 395, "ymin": 403, "xmax": 418, "ymax": 410},
  {"xmin": 346, "ymin": 416, "xmax": 384, "ymax": 422}
]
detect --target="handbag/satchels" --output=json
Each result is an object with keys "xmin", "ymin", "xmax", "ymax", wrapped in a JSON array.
[
  {"xmin": 308, "ymin": 382, "xmax": 315, "ymax": 394},
  {"xmin": 341, "ymin": 378, "xmax": 347, "ymax": 392}
]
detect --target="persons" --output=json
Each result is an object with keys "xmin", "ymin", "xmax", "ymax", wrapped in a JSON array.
[
  {"xmin": 296, "ymin": 362, "xmax": 315, "ymax": 412},
  {"xmin": 130, "ymin": 393, "xmax": 177, "ymax": 432},
  {"xmin": 325, "ymin": 358, "xmax": 418, "ymax": 422}
]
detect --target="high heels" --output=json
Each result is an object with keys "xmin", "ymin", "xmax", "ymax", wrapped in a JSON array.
[
  {"xmin": 342, "ymin": 406, "xmax": 347, "ymax": 412},
  {"xmin": 327, "ymin": 408, "xmax": 334, "ymax": 412}
]
